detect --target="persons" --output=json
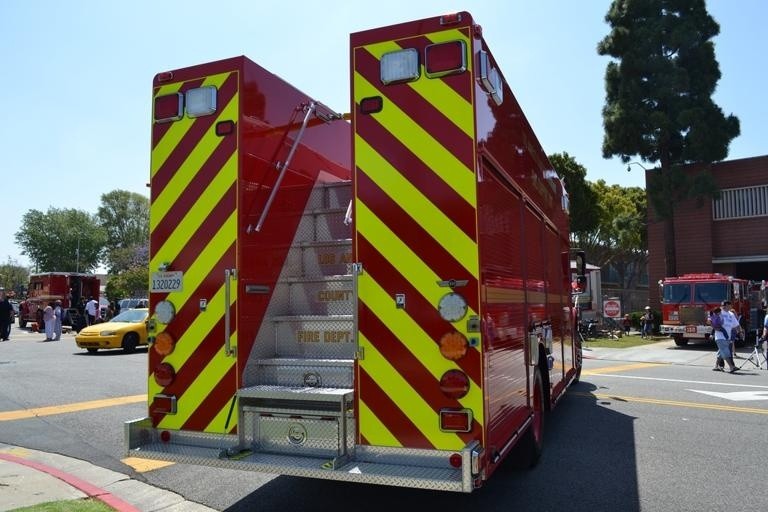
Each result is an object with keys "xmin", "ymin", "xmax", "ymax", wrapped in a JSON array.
[
  {"xmin": 0, "ymin": 284, "xmax": 120, "ymax": 342},
  {"xmin": 643, "ymin": 306, "xmax": 654, "ymax": 340},
  {"xmin": 136, "ymin": 301, "xmax": 145, "ymax": 308},
  {"xmin": 710, "ymin": 280, "xmax": 768, "ymax": 373},
  {"xmin": 623, "ymin": 313, "xmax": 633, "ymax": 336},
  {"xmin": 640, "ymin": 312, "xmax": 647, "ymax": 338}
]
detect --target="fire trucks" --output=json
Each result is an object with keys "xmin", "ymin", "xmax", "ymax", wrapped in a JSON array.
[
  {"xmin": 658, "ymin": 273, "xmax": 768, "ymax": 351},
  {"xmin": 122, "ymin": 10, "xmax": 587, "ymax": 495}
]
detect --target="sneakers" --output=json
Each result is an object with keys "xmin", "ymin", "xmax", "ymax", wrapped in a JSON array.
[{"xmin": 712, "ymin": 367, "xmax": 740, "ymax": 373}]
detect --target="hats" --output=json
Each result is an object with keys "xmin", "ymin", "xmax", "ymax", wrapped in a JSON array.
[
  {"xmin": 722, "ymin": 301, "xmax": 731, "ymax": 305},
  {"xmin": 644, "ymin": 306, "xmax": 651, "ymax": 310},
  {"xmin": 56, "ymin": 300, "xmax": 62, "ymax": 303}
]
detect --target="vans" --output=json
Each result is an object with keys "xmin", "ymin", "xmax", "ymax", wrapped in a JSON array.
[{"xmin": 19, "ymin": 271, "xmax": 101, "ymax": 333}]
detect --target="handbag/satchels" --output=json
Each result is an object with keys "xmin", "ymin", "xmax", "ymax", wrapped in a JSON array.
[
  {"xmin": 51, "ymin": 314, "xmax": 56, "ymax": 320},
  {"xmin": 9, "ymin": 310, "xmax": 14, "ymax": 323},
  {"xmin": 644, "ymin": 325, "xmax": 647, "ymax": 331}
]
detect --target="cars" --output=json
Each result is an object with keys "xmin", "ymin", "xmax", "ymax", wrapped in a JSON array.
[{"xmin": 74, "ymin": 297, "xmax": 149, "ymax": 355}]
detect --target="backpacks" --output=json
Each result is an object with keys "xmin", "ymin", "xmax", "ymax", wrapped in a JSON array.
[{"xmin": 61, "ymin": 309, "xmax": 65, "ymax": 321}]
